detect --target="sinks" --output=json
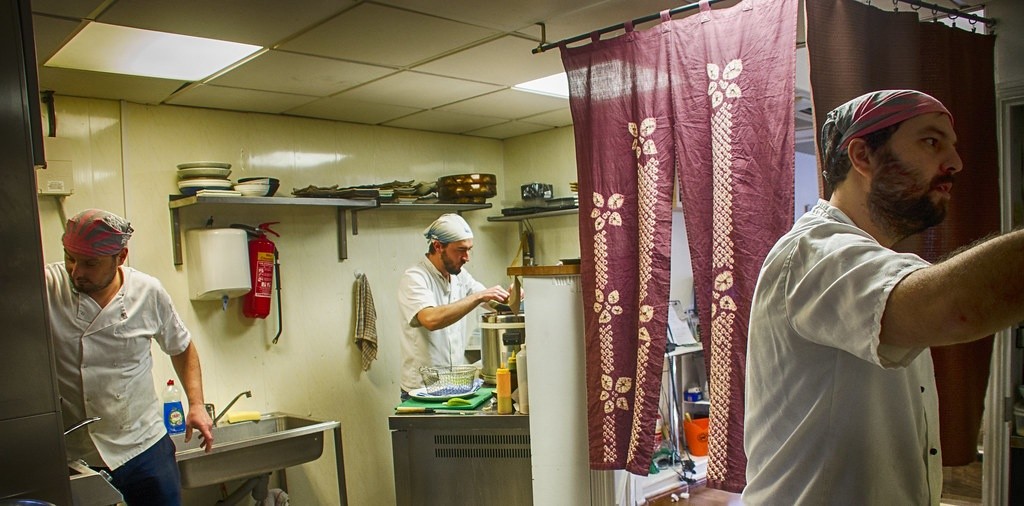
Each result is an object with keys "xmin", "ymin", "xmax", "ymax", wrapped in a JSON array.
[{"xmin": 170, "ymin": 411, "xmax": 342, "ymax": 489}]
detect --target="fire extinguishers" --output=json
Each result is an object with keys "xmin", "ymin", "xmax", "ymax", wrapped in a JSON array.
[{"xmin": 230, "ymin": 220, "xmax": 282, "ymax": 320}]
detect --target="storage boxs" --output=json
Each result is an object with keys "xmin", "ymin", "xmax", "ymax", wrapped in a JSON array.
[{"xmin": 521, "ymin": 183, "xmax": 553, "ymax": 199}]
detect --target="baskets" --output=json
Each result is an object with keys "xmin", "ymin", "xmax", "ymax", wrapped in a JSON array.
[{"xmin": 418, "ymin": 365, "xmax": 477, "ymax": 395}]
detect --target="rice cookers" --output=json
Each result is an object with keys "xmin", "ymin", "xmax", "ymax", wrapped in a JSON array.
[{"xmin": 480, "ymin": 311, "xmax": 524, "ymax": 384}]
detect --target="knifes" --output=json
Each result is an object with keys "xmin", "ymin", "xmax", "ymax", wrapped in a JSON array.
[{"xmin": 396, "ymin": 407, "xmax": 483, "ymax": 414}]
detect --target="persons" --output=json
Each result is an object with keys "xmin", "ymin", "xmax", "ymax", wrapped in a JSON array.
[
  {"xmin": 44, "ymin": 210, "xmax": 214, "ymax": 506},
  {"xmin": 737, "ymin": 89, "xmax": 1024, "ymax": 506},
  {"xmin": 395, "ymin": 213, "xmax": 524, "ymax": 403}
]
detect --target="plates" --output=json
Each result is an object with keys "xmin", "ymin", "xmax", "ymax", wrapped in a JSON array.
[
  {"xmin": 408, "ymin": 387, "xmax": 476, "ymax": 402},
  {"xmin": 567, "ymin": 182, "xmax": 579, "ymax": 206},
  {"xmin": 291, "ymin": 178, "xmax": 418, "ymax": 203},
  {"xmin": 176, "ymin": 160, "xmax": 232, "ymax": 196}
]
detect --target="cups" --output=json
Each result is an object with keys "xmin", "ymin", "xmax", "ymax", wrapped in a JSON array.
[{"xmin": 0, "ymin": 499, "xmax": 56, "ymax": 506}]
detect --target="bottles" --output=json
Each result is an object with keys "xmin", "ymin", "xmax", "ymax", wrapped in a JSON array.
[
  {"xmin": 653, "ymin": 415, "xmax": 664, "ymax": 452},
  {"xmin": 496, "ymin": 343, "xmax": 529, "ymax": 415},
  {"xmin": 161, "ymin": 378, "xmax": 186, "ymax": 435}
]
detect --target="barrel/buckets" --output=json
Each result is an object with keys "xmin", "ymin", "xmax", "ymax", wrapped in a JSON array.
[{"xmin": 679, "ymin": 415, "xmax": 710, "ymax": 457}]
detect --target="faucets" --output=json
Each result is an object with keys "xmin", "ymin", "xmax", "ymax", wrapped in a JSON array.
[{"xmin": 205, "ymin": 390, "xmax": 252, "ymax": 428}]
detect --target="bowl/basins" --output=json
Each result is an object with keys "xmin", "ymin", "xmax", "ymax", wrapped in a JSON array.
[
  {"xmin": 234, "ymin": 177, "xmax": 281, "ymax": 198},
  {"xmin": 520, "ymin": 181, "xmax": 553, "ymax": 200},
  {"xmin": 419, "ymin": 364, "xmax": 477, "ymax": 394}
]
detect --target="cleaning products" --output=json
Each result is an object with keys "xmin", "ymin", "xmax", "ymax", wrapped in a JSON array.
[{"xmin": 162, "ymin": 378, "xmax": 185, "ymax": 435}]
